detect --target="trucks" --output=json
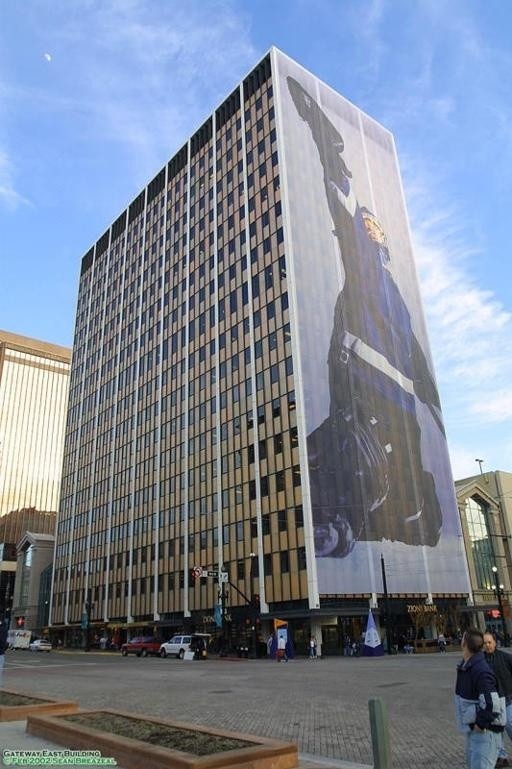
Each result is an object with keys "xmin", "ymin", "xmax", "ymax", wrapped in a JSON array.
[{"xmin": 7, "ymin": 630, "xmax": 32, "ymax": 649}]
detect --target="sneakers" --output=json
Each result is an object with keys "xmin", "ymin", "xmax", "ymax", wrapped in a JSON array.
[{"xmin": 495, "ymin": 757, "xmax": 508, "ymax": 768}]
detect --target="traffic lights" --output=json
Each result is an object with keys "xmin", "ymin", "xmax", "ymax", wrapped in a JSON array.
[
  {"xmin": 252, "ymin": 594, "xmax": 259, "ymax": 609},
  {"xmin": 245, "ymin": 617, "xmax": 253, "ymax": 626},
  {"xmin": 255, "ymin": 615, "xmax": 261, "ymax": 625}
]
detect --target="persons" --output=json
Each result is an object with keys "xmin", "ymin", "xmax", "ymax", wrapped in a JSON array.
[
  {"xmin": 306, "ymin": 108, "xmax": 446, "ymax": 559},
  {"xmin": 453, "ymin": 628, "xmax": 507, "ymax": 769},
  {"xmin": 0, "ymin": 615, "xmax": 10, "ymax": 676},
  {"xmin": 195, "ymin": 638, "xmax": 249, "ymax": 660},
  {"xmin": 438, "ymin": 630, "xmax": 464, "ymax": 655},
  {"xmin": 391, "ymin": 626, "xmax": 427, "ymax": 656},
  {"xmin": 486, "ymin": 628, "xmax": 512, "ymax": 649},
  {"xmin": 266, "ymin": 632, "xmax": 288, "ymax": 663},
  {"xmin": 343, "ymin": 633, "xmax": 361, "ymax": 657},
  {"xmin": 308, "ymin": 633, "xmax": 317, "ymax": 660},
  {"xmin": 481, "ymin": 630, "xmax": 512, "ymax": 769}
]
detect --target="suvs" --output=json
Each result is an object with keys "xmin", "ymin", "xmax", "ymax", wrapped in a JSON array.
[
  {"xmin": 122, "ymin": 637, "xmax": 162, "ymax": 657},
  {"xmin": 158, "ymin": 633, "xmax": 212, "ymax": 659}
]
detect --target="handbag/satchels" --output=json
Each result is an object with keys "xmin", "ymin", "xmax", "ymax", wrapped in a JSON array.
[{"xmin": 277, "ymin": 650, "xmax": 284, "ymax": 657}]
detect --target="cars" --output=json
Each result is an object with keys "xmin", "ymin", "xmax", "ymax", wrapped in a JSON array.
[{"xmin": 29, "ymin": 639, "xmax": 52, "ymax": 652}]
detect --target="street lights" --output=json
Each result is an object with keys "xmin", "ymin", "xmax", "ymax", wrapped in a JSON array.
[{"xmin": 490, "ymin": 567, "xmax": 511, "ymax": 646}]
